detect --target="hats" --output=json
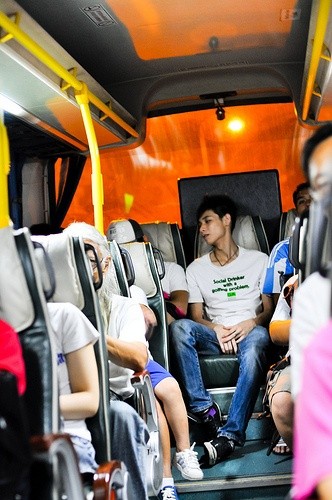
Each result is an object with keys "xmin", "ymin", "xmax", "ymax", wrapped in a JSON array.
[{"xmin": 105, "ymin": 217, "xmax": 144, "ymax": 245}]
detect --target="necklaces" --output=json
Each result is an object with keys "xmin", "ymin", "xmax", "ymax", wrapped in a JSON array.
[{"xmin": 213, "ymin": 247, "xmax": 238, "ymax": 265}]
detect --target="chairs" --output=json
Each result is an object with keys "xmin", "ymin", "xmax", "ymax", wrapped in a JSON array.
[{"xmin": 0, "ymin": 182, "xmax": 331, "ymax": 500}]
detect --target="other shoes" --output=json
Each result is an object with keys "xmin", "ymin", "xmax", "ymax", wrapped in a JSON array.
[
  {"xmin": 273, "ymin": 438, "xmax": 291, "ymax": 456},
  {"xmin": 204, "ymin": 436, "xmax": 234, "ymax": 465},
  {"xmin": 198, "ymin": 402, "xmax": 223, "ymax": 439}
]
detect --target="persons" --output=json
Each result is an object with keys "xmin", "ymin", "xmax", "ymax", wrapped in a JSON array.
[
  {"xmin": 128, "ymin": 285, "xmax": 204, "ymax": 500},
  {"xmin": 269, "ymin": 275, "xmax": 306, "ymax": 451},
  {"xmin": 63, "ymin": 222, "xmax": 150, "ymax": 500},
  {"xmin": 47, "ymin": 302, "xmax": 100, "ymax": 500},
  {"xmin": 168, "ymin": 195, "xmax": 273, "ymax": 465},
  {"xmin": 129, "ymin": 219, "xmax": 189, "ymax": 326},
  {"xmin": 262, "ymin": 182, "xmax": 311, "ymax": 455},
  {"xmin": 304, "ymin": 120, "xmax": 332, "ymax": 215},
  {"xmin": 290, "ymin": 270, "xmax": 332, "ymax": 499}
]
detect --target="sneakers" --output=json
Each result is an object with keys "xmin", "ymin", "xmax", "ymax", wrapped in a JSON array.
[
  {"xmin": 160, "ymin": 484, "xmax": 179, "ymax": 500},
  {"xmin": 172, "ymin": 441, "xmax": 204, "ymax": 481}
]
